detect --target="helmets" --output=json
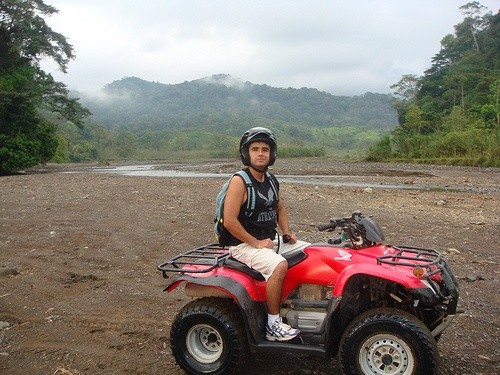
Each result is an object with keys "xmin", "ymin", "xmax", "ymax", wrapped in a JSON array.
[{"xmin": 239, "ymin": 127, "xmax": 277, "ymax": 165}]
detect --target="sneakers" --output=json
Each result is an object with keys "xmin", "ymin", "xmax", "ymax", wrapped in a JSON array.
[{"xmin": 266, "ymin": 316, "xmax": 300, "ymax": 342}]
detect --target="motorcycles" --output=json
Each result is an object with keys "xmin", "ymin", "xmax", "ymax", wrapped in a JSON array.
[{"xmin": 158, "ymin": 210, "xmax": 466, "ymax": 375}]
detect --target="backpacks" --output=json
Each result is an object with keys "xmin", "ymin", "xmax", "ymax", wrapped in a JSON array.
[{"xmin": 213, "ymin": 171, "xmax": 279, "ymax": 247}]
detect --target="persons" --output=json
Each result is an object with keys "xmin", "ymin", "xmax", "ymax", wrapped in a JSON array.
[{"xmin": 218, "ymin": 127, "xmax": 311, "ymax": 341}]
